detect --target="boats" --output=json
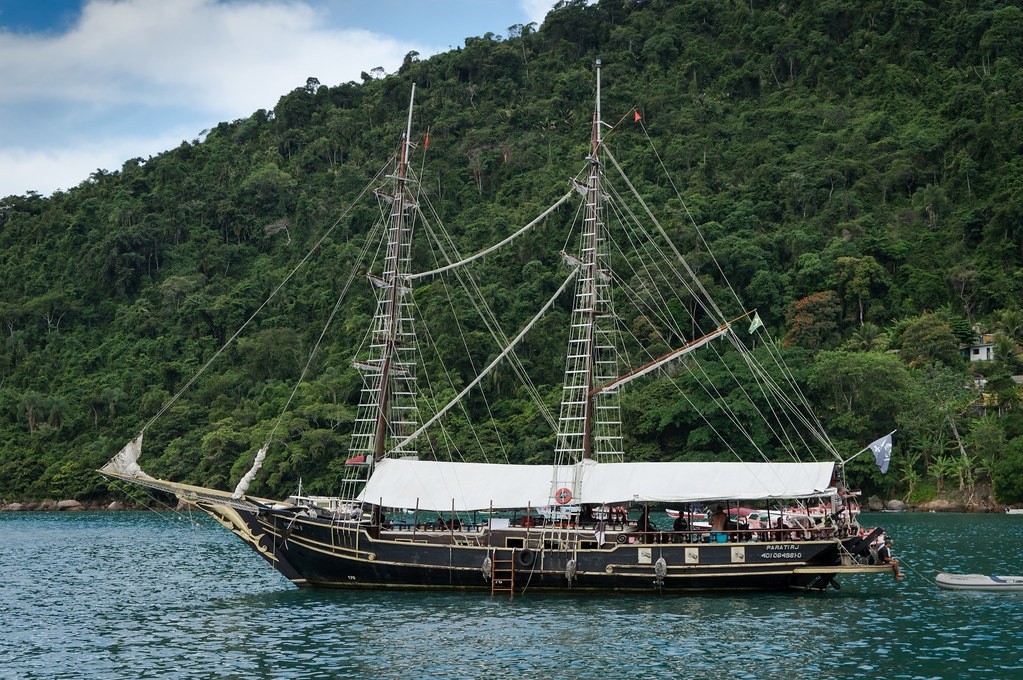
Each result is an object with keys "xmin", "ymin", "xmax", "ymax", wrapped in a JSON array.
[
  {"xmin": 1005, "ymin": 508, "xmax": 1023, "ymax": 515},
  {"xmin": 934, "ymin": 571, "xmax": 1023, "ymax": 590}
]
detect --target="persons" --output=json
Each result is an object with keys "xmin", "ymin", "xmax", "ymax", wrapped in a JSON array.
[
  {"xmin": 774, "ymin": 517, "xmax": 791, "ymax": 541},
  {"xmin": 673, "ymin": 511, "xmax": 688, "ymax": 531},
  {"xmin": 878, "ymin": 536, "xmax": 905, "ymax": 581},
  {"xmin": 709, "ymin": 506, "xmax": 727, "ymax": 543},
  {"xmin": 373, "ymin": 507, "xmax": 387, "ymax": 528},
  {"xmin": 638, "ymin": 506, "xmax": 659, "ymax": 533}
]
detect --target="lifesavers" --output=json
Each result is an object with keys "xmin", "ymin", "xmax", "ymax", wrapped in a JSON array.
[
  {"xmin": 555, "ymin": 488, "xmax": 572, "ymax": 503},
  {"xmin": 518, "ymin": 549, "xmax": 535, "ymax": 567}
]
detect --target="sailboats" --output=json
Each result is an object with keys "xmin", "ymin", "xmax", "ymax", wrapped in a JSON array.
[{"xmin": 94, "ymin": 55, "xmax": 905, "ymax": 594}]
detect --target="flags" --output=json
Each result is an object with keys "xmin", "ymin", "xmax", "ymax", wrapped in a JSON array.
[
  {"xmin": 870, "ymin": 434, "xmax": 892, "ymax": 474},
  {"xmin": 748, "ymin": 311, "xmax": 763, "ymax": 335}
]
